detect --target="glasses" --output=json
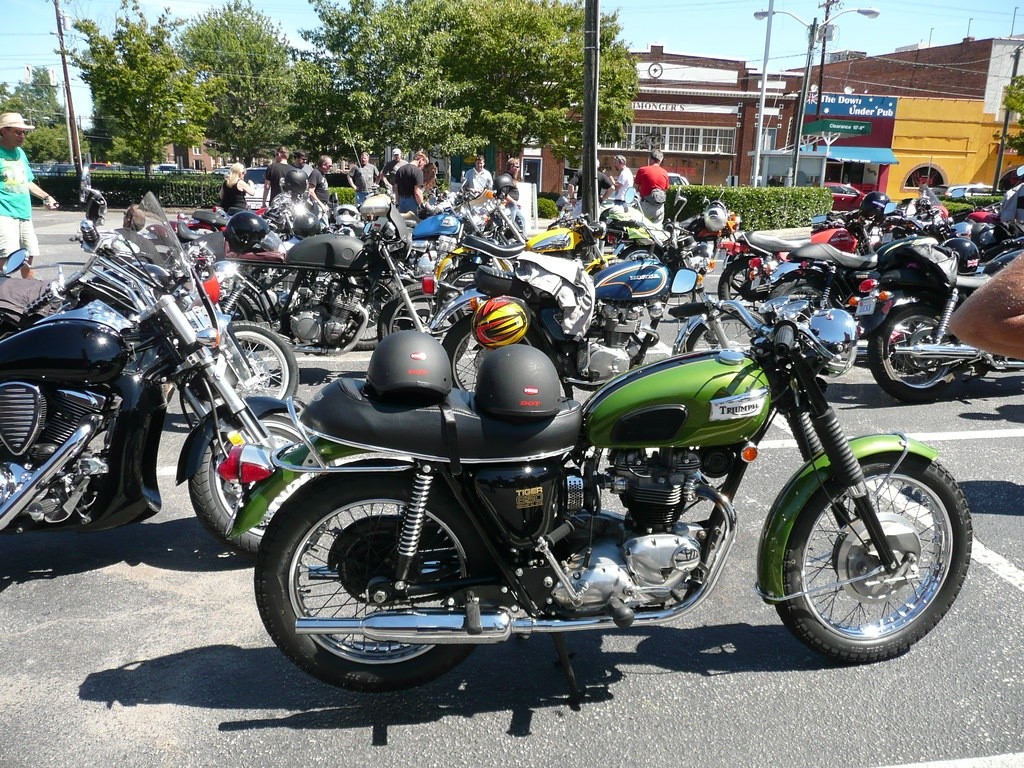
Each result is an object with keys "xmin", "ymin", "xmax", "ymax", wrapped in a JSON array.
[{"xmin": 6, "ymin": 127, "xmax": 29, "ymax": 135}]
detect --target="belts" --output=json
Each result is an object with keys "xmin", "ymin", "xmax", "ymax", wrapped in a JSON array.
[{"xmin": 641, "ymin": 199, "xmax": 649, "ymax": 202}]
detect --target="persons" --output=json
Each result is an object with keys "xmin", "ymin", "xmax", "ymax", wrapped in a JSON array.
[
  {"xmin": 347, "ymin": 152, "xmax": 381, "ymax": 208},
  {"xmin": 555, "ymin": 191, "xmax": 569, "ymax": 211},
  {"xmin": 395, "ymin": 150, "xmax": 429, "ymax": 216},
  {"xmin": 948, "ymin": 251, "xmax": 1024, "ymax": 362},
  {"xmin": 219, "ymin": 162, "xmax": 255, "ymax": 212},
  {"xmin": 0, "ymin": 112, "xmax": 60, "ymax": 281},
  {"xmin": 308, "ymin": 155, "xmax": 333, "ymax": 227},
  {"xmin": 568, "ymin": 157, "xmax": 616, "ymax": 219},
  {"xmin": 502, "ymin": 158, "xmax": 529, "ymax": 242},
  {"xmin": 609, "ymin": 155, "xmax": 634, "ymax": 205},
  {"xmin": 261, "ymin": 145, "xmax": 296, "ymax": 209},
  {"xmin": 380, "ymin": 148, "xmax": 409, "ymax": 202},
  {"xmin": 634, "ymin": 149, "xmax": 669, "ymax": 224},
  {"xmin": 288, "ymin": 149, "xmax": 314, "ymax": 177},
  {"xmin": 463, "ymin": 155, "xmax": 494, "ymax": 196}
]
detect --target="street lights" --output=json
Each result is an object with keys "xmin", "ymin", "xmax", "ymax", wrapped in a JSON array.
[{"xmin": 754, "ymin": 9, "xmax": 879, "ymax": 188}]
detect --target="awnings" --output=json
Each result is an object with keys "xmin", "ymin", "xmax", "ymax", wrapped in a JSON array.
[{"xmin": 800, "ymin": 145, "xmax": 900, "ymax": 165}]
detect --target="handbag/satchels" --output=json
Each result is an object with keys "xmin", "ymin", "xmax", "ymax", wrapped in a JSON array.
[{"xmin": 646, "ymin": 189, "xmax": 666, "ymax": 205}]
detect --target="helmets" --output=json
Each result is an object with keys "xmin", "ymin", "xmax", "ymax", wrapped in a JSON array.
[
  {"xmin": 860, "ymin": 192, "xmax": 891, "ymax": 224},
  {"xmin": 294, "ymin": 212, "xmax": 320, "ymax": 238},
  {"xmin": 336, "ymin": 203, "xmax": 361, "ymax": 226},
  {"xmin": 473, "ymin": 344, "xmax": 561, "ymax": 416},
  {"xmin": 223, "ymin": 212, "xmax": 270, "ymax": 251},
  {"xmin": 285, "ymin": 169, "xmax": 309, "ymax": 195},
  {"xmin": 470, "ymin": 295, "xmax": 531, "ymax": 349},
  {"xmin": 704, "ymin": 202, "xmax": 727, "ymax": 232},
  {"xmin": 493, "ymin": 175, "xmax": 516, "ymax": 192},
  {"xmin": 940, "ymin": 238, "xmax": 981, "ymax": 275},
  {"xmin": 366, "ymin": 331, "xmax": 453, "ymax": 396}
]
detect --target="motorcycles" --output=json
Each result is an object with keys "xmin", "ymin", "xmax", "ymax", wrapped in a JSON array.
[
  {"xmin": 0, "ymin": 165, "xmax": 1024, "ymax": 426},
  {"xmin": 212, "ymin": 266, "xmax": 975, "ymax": 692},
  {"xmin": 0, "ymin": 190, "xmax": 338, "ymax": 555}
]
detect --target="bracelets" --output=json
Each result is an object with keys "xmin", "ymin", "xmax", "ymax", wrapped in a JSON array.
[
  {"xmin": 43, "ymin": 194, "xmax": 51, "ymax": 201},
  {"xmin": 570, "ymin": 198, "xmax": 575, "ymax": 199}
]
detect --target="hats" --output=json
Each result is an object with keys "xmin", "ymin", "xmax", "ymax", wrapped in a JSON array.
[
  {"xmin": 393, "ymin": 149, "xmax": 401, "ymax": 155},
  {"xmin": 0, "ymin": 113, "xmax": 36, "ymax": 130}
]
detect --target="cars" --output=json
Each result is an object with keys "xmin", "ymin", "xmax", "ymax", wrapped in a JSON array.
[
  {"xmin": 668, "ymin": 173, "xmax": 689, "ymax": 185},
  {"xmin": 89, "ymin": 163, "xmax": 108, "ymax": 171},
  {"xmin": 158, "ymin": 164, "xmax": 178, "ymax": 173},
  {"xmin": 930, "ymin": 185, "xmax": 1000, "ymax": 197},
  {"xmin": 824, "ymin": 183, "xmax": 866, "ymax": 212},
  {"xmin": 243, "ymin": 167, "xmax": 271, "ymax": 210}
]
